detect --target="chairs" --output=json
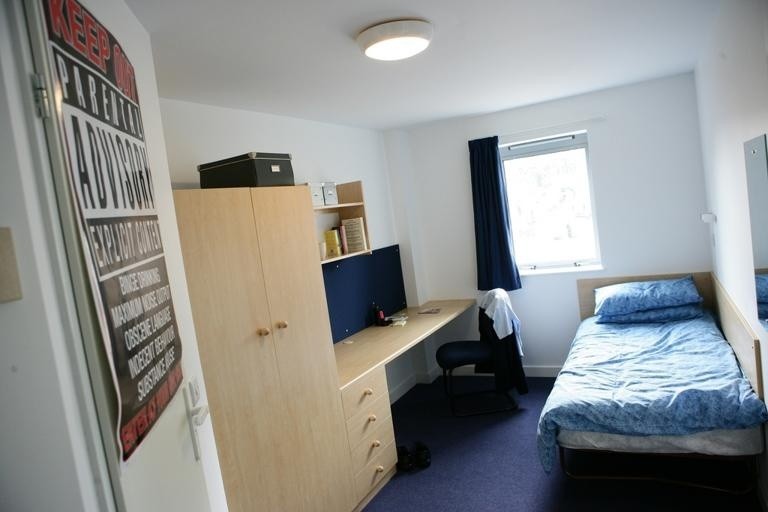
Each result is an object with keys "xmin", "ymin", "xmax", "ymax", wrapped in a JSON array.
[{"xmin": 436, "ymin": 289, "xmax": 525, "ymax": 417}]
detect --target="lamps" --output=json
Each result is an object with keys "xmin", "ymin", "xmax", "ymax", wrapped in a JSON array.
[
  {"xmin": 355, "ymin": 17, "xmax": 434, "ymax": 61},
  {"xmin": 508, "ymin": 136, "xmax": 575, "ymax": 151}
]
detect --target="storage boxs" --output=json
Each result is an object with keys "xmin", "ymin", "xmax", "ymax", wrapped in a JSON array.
[{"xmin": 196, "ymin": 151, "xmax": 295, "ymax": 187}]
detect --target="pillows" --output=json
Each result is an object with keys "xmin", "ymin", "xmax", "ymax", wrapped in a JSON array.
[
  {"xmin": 593, "ymin": 304, "xmax": 702, "ymax": 324},
  {"xmin": 592, "ymin": 273, "xmax": 703, "ymax": 315}
]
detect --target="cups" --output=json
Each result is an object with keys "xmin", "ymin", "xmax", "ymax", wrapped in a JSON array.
[{"xmin": 319, "ymin": 241, "xmax": 326, "ymax": 261}]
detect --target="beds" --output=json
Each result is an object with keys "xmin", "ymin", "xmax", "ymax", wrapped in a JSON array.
[{"xmin": 536, "ymin": 272, "xmax": 768, "ymax": 500}]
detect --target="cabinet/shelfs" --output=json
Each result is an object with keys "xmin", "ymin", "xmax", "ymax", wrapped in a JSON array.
[
  {"xmin": 340, "ymin": 363, "xmax": 398, "ymax": 502},
  {"xmin": 172, "ymin": 180, "xmax": 373, "ymax": 511}
]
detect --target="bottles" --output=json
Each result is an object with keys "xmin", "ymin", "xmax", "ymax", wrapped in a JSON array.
[{"xmin": 373, "ymin": 302, "xmax": 385, "ymax": 327}]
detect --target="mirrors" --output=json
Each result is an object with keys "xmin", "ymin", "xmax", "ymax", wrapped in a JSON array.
[{"xmin": 742, "ymin": 134, "xmax": 768, "ymax": 331}]
